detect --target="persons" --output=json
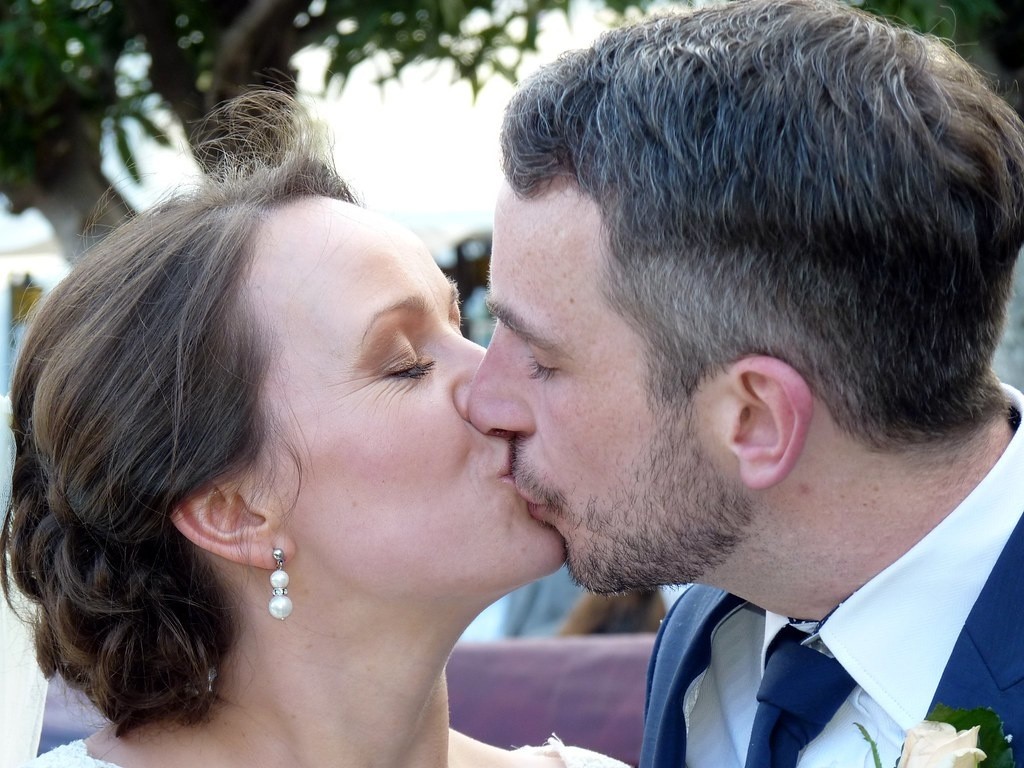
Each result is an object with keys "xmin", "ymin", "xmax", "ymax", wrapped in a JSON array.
[
  {"xmin": 467, "ymin": 0, "xmax": 1024, "ymax": 768},
  {"xmin": 0, "ymin": 68, "xmax": 634, "ymax": 768}
]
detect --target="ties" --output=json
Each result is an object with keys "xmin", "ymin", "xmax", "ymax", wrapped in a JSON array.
[{"xmin": 746, "ymin": 634, "xmax": 856, "ymax": 768}]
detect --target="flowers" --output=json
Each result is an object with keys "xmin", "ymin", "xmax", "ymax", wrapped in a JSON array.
[{"xmin": 852, "ymin": 702, "xmax": 1016, "ymax": 768}]
